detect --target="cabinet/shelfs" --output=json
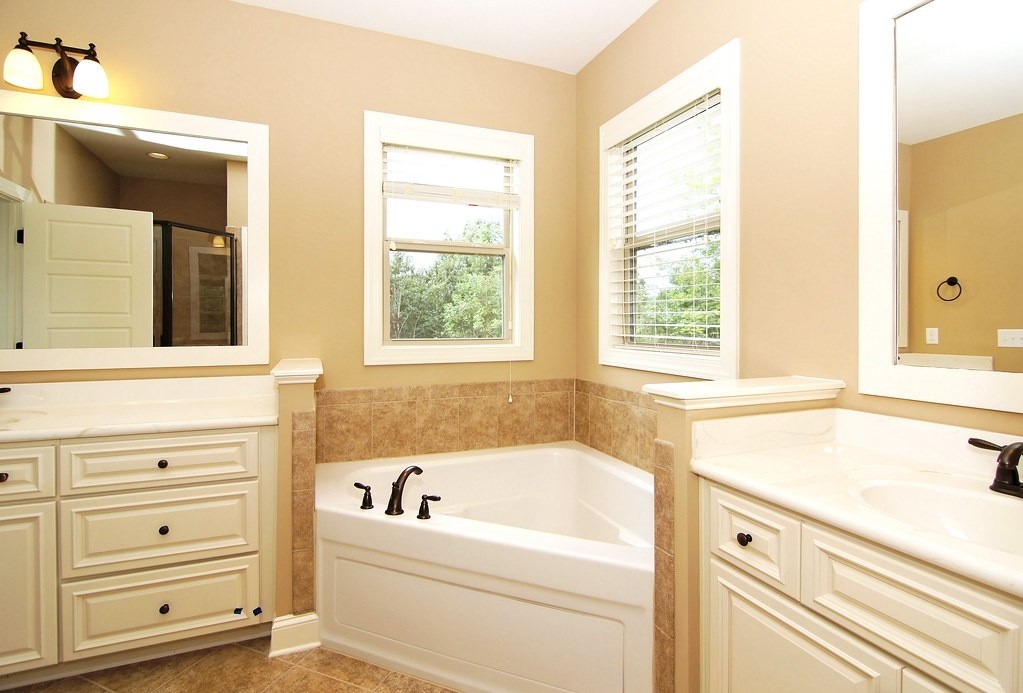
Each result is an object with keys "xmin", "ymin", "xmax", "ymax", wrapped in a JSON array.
[
  {"xmin": 0, "ymin": 432, "xmax": 260, "ymax": 676},
  {"xmin": 705, "ymin": 487, "xmax": 1023, "ymax": 693}
]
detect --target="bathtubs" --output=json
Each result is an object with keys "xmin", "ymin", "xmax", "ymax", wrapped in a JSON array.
[{"xmin": 313, "ymin": 440, "xmax": 655, "ymax": 693}]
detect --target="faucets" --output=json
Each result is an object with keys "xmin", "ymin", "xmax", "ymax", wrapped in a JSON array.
[
  {"xmin": 384, "ymin": 466, "xmax": 424, "ymax": 516},
  {"xmin": 988, "ymin": 437, "xmax": 1023, "ymax": 501}
]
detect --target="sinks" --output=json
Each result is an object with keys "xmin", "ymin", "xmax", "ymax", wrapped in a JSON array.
[
  {"xmin": 0, "ymin": 409, "xmax": 48, "ymax": 426},
  {"xmin": 859, "ymin": 478, "xmax": 1023, "ymax": 561}
]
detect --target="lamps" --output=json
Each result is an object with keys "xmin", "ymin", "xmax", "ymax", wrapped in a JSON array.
[
  {"xmin": 3, "ymin": 32, "xmax": 110, "ymax": 100},
  {"xmin": 211, "ymin": 236, "xmax": 224, "ymax": 248}
]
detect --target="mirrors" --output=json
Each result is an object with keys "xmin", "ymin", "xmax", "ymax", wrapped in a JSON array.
[
  {"xmin": 859, "ymin": 0, "xmax": 1023, "ymax": 415},
  {"xmin": 0, "ymin": 89, "xmax": 270, "ymax": 372}
]
